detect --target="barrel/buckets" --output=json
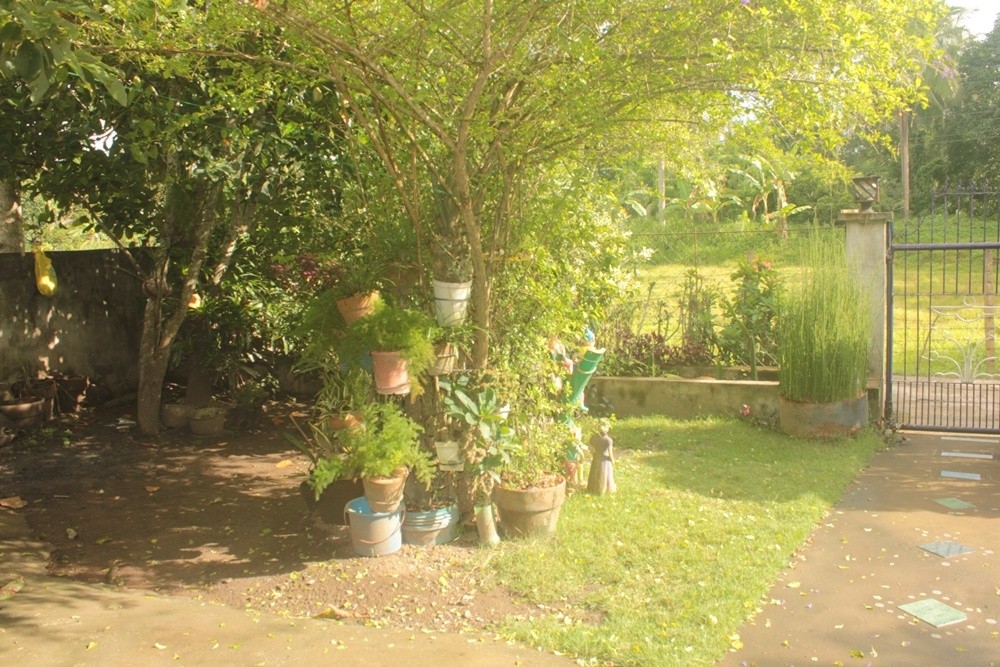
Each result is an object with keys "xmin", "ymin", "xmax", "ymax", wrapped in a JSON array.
[
  {"xmin": 343, "ymin": 496, "xmax": 407, "ymax": 557},
  {"xmin": 161, "ymin": 403, "xmax": 226, "ymax": 438}
]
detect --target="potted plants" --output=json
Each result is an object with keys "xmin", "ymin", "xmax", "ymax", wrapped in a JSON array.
[
  {"xmin": 0, "ymin": 363, "xmax": 89, "ymax": 428},
  {"xmin": 161, "ymin": 248, "xmax": 599, "ymax": 548},
  {"xmin": 771, "ymin": 214, "xmax": 870, "ymax": 438}
]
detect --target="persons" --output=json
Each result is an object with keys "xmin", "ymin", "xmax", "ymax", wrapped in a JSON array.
[
  {"xmin": 587, "ymin": 424, "xmax": 617, "ymax": 495},
  {"xmin": 562, "ymin": 426, "xmax": 587, "ymax": 485}
]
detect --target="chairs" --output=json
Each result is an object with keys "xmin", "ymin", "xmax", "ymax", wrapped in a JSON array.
[{"xmin": 561, "ymin": 344, "xmax": 607, "ymax": 461}]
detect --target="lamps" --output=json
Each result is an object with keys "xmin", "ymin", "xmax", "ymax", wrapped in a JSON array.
[{"xmin": 851, "ymin": 175, "xmax": 881, "ymax": 215}]
